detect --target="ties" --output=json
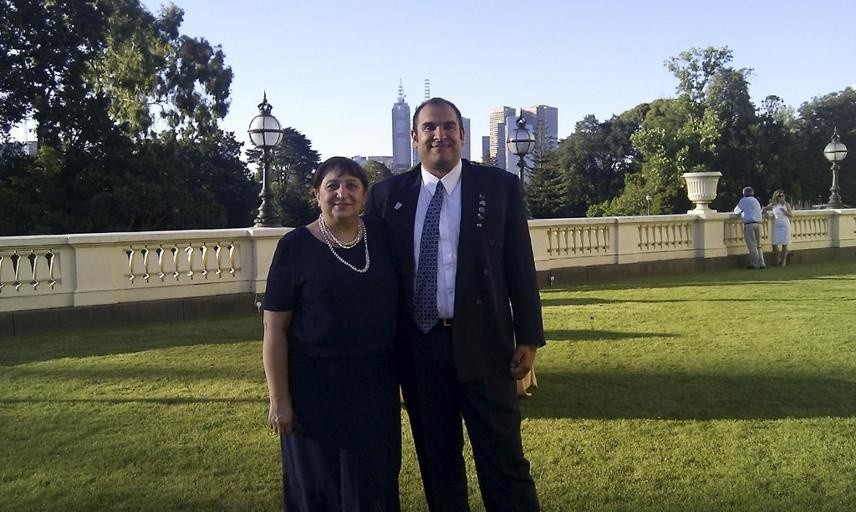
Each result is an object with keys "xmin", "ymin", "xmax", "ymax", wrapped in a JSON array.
[{"xmin": 412, "ymin": 181, "xmax": 444, "ymax": 335}]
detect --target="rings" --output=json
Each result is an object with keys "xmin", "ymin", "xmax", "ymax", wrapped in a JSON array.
[{"xmin": 512, "ymin": 359, "xmax": 520, "ymax": 365}]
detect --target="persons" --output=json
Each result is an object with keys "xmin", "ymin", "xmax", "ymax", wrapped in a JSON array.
[
  {"xmin": 734, "ymin": 187, "xmax": 768, "ymax": 269},
  {"xmin": 762, "ymin": 189, "xmax": 795, "ymax": 268},
  {"xmin": 257, "ymin": 155, "xmax": 404, "ymax": 512},
  {"xmin": 364, "ymin": 97, "xmax": 548, "ymax": 510}
]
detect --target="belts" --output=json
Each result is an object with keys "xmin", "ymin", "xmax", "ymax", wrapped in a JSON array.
[
  {"xmin": 435, "ymin": 318, "xmax": 454, "ymax": 329},
  {"xmin": 745, "ymin": 222, "xmax": 757, "ymax": 225}
]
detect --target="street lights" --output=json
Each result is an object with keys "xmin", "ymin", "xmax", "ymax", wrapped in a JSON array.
[
  {"xmin": 247, "ymin": 88, "xmax": 291, "ymax": 234},
  {"xmin": 645, "ymin": 192, "xmax": 652, "ymax": 215},
  {"xmin": 821, "ymin": 126, "xmax": 849, "ymax": 208},
  {"xmin": 507, "ymin": 108, "xmax": 539, "ymax": 220}
]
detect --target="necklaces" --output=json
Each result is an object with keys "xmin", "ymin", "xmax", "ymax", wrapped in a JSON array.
[{"xmin": 319, "ymin": 207, "xmax": 371, "ymax": 275}]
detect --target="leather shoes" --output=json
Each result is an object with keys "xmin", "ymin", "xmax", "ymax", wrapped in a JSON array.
[{"xmin": 747, "ymin": 266, "xmax": 755, "ymax": 269}]
